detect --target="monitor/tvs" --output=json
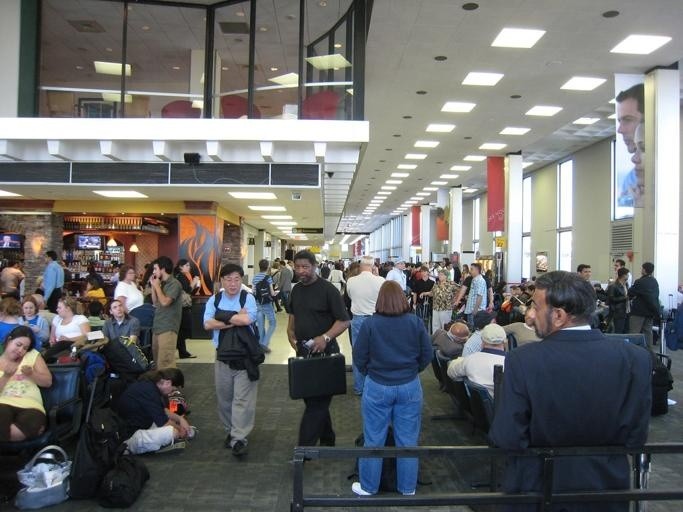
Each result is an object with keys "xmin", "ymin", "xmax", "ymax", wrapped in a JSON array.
[
  {"xmin": 0, "ymin": 234, "xmax": 24, "ymax": 250},
  {"xmin": 74, "ymin": 234, "xmax": 105, "ymax": 250}
]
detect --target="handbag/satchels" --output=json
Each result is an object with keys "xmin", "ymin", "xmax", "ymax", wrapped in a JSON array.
[
  {"xmin": 78, "ymin": 349, "xmax": 110, "ymax": 406},
  {"xmin": 347, "ymin": 423, "xmax": 397, "ymax": 492},
  {"xmin": 15, "ymin": 445, "xmax": 72, "ymax": 510},
  {"xmin": 98, "ymin": 443, "xmax": 150, "ymax": 508},
  {"xmin": 181, "ymin": 290, "xmax": 194, "ymax": 311},
  {"xmin": 639, "ymin": 342, "xmax": 673, "ymax": 416}
]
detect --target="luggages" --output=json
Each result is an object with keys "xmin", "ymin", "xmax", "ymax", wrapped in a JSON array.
[{"xmin": 664, "ymin": 293, "xmax": 683, "ymax": 351}]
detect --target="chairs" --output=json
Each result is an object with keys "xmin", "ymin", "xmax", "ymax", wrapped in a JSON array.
[
  {"xmin": 428, "ymin": 344, "xmax": 504, "ymax": 492},
  {"xmin": 603, "ymin": 333, "xmax": 672, "ymax": 372},
  {"xmin": 0, "ymin": 326, "xmax": 153, "ymax": 466}
]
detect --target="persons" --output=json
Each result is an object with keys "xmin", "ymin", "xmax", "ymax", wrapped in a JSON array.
[
  {"xmin": 630, "ymin": 110, "xmax": 647, "ymax": 206},
  {"xmin": 492, "ymin": 269, "xmax": 652, "ymax": 510},
  {"xmin": 615, "ymin": 79, "xmax": 644, "ymax": 203}
]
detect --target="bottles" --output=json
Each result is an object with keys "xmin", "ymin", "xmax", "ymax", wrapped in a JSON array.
[
  {"xmin": 62, "ymin": 216, "xmax": 142, "ymax": 231},
  {"xmin": 62, "ymin": 250, "xmax": 121, "ymax": 273},
  {"xmin": 70, "ymin": 346, "xmax": 78, "ymax": 362}
]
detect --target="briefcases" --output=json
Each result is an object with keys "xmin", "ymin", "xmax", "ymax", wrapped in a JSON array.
[{"xmin": 287, "ymin": 347, "xmax": 347, "ymax": 399}]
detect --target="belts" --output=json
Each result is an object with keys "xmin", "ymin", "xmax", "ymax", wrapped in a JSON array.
[{"xmin": 296, "ymin": 339, "xmax": 308, "ymax": 342}]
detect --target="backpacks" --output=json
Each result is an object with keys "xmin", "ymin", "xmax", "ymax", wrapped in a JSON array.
[
  {"xmin": 67, "ymin": 407, "xmax": 118, "ymax": 501},
  {"xmin": 255, "ymin": 275, "xmax": 273, "ymax": 305},
  {"xmin": 101, "ymin": 336, "xmax": 148, "ymax": 374}
]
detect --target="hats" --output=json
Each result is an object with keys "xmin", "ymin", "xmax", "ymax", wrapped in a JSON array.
[
  {"xmin": 473, "ymin": 310, "xmax": 498, "ymax": 328},
  {"xmin": 479, "ymin": 323, "xmax": 506, "ymax": 344},
  {"xmin": 393, "ymin": 257, "xmax": 406, "ymax": 265}
]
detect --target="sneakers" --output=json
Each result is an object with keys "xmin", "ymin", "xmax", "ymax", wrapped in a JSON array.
[
  {"xmin": 224, "ymin": 433, "xmax": 248, "ymax": 455},
  {"xmin": 189, "ymin": 425, "xmax": 198, "ymax": 439},
  {"xmin": 351, "ymin": 481, "xmax": 371, "ymax": 496}
]
